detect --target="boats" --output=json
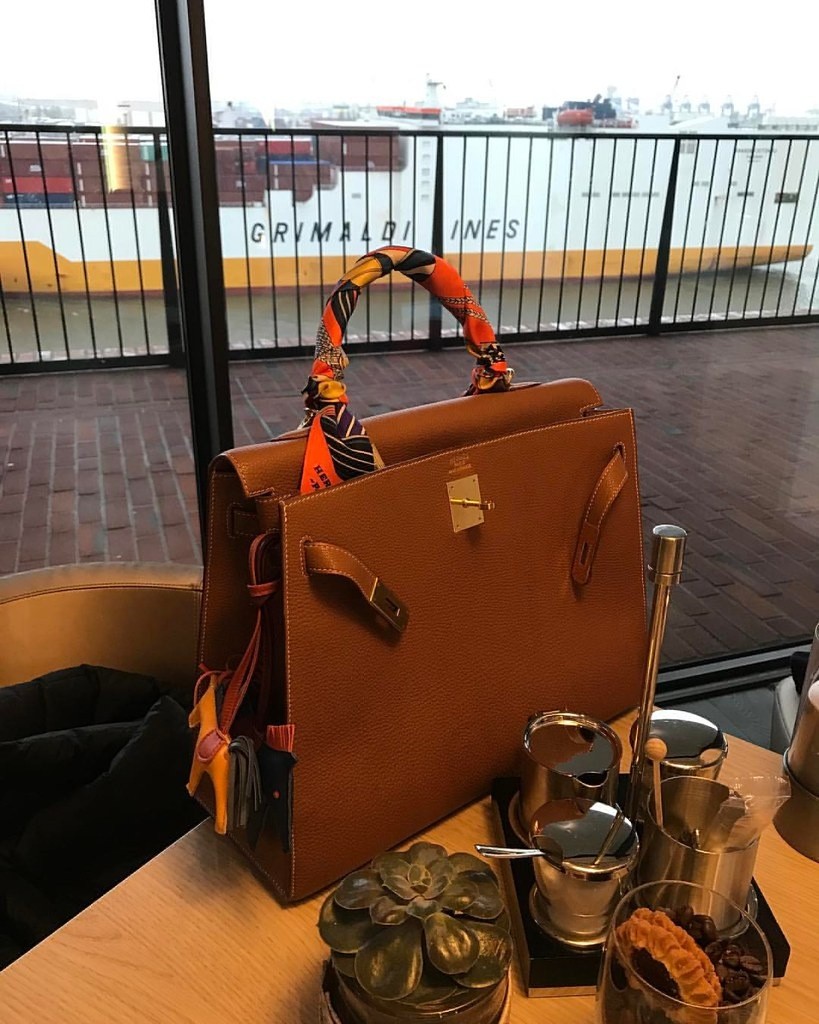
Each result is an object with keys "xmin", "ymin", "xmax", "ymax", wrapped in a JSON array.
[{"xmin": 0, "ymin": 120, "xmax": 819, "ymax": 294}]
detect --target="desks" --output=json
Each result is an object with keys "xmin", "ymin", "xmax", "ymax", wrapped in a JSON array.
[{"xmin": 0, "ymin": 699, "xmax": 818, "ymax": 1024}]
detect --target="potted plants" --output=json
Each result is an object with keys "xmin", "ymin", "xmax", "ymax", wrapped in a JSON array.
[{"xmin": 316, "ymin": 839, "xmax": 514, "ymax": 1024}]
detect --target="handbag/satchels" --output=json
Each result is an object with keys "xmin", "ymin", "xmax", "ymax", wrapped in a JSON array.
[{"xmin": 184, "ymin": 246, "xmax": 649, "ymax": 902}]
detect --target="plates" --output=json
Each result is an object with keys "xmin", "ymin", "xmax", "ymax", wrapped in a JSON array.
[{"xmin": 509, "ymin": 791, "xmax": 537, "ymax": 848}]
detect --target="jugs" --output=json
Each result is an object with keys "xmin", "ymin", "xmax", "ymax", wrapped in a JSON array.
[{"xmin": 522, "ymin": 709, "xmax": 622, "ymax": 837}]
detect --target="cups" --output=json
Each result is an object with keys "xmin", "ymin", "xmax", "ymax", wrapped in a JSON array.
[
  {"xmin": 640, "ymin": 777, "xmax": 759, "ymax": 928},
  {"xmin": 530, "ymin": 798, "xmax": 633, "ymax": 942},
  {"xmin": 631, "ymin": 708, "xmax": 727, "ymax": 818},
  {"xmin": 597, "ymin": 882, "xmax": 773, "ymax": 1024},
  {"xmin": 783, "ymin": 623, "xmax": 819, "ymax": 800}
]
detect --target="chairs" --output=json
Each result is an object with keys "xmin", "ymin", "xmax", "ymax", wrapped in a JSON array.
[{"xmin": 0, "ymin": 562, "xmax": 206, "ymax": 692}]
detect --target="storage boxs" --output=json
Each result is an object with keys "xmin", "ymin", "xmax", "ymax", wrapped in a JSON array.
[{"xmin": 0, "ymin": 117, "xmax": 405, "ymax": 209}]
